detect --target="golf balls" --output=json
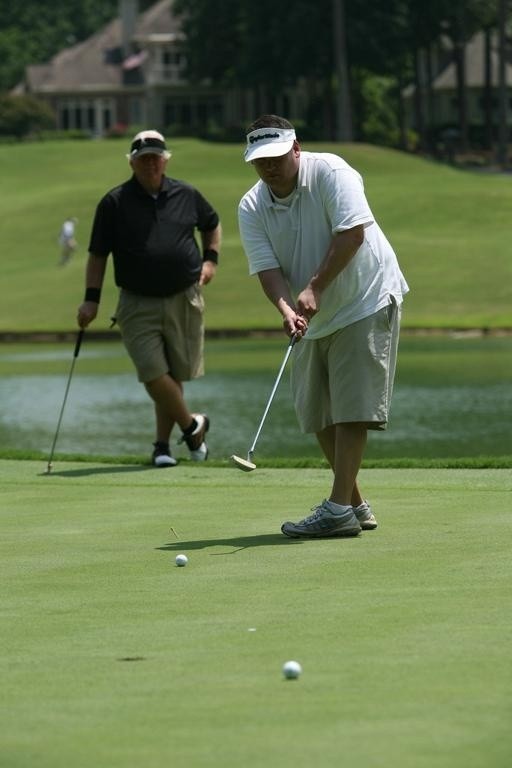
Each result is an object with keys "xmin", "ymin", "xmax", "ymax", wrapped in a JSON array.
[
  {"xmin": 282, "ymin": 662, "xmax": 299, "ymax": 678},
  {"xmin": 175, "ymin": 554, "xmax": 186, "ymax": 567}
]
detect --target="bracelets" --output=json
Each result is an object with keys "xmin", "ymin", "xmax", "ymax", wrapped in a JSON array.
[
  {"xmin": 202, "ymin": 247, "xmax": 220, "ymax": 265},
  {"xmin": 83, "ymin": 288, "xmax": 102, "ymax": 307}
]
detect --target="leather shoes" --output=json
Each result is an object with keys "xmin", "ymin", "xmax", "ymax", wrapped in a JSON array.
[
  {"xmin": 178, "ymin": 412, "xmax": 209, "ymax": 459},
  {"xmin": 151, "ymin": 442, "xmax": 176, "ymax": 466}
]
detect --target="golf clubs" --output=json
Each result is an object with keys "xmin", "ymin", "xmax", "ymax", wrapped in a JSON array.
[
  {"xmin": 48, "ymin": 326, "xmax": 82, "ymax": 473},
  {"xmin": 232, "ymin": 314, "xmax": 303, "ymax": 470}
]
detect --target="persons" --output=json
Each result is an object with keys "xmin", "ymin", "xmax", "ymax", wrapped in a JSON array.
[
  {"xmin": 236, "ymin": 115, "xmax": 410, "ymax": 538},
  {"xmin": 76, "ymin": 128, "xmax": 223, "ymax": 464},
  {"xmin": 59, "ymin": 215, "xmax": 79, "ymax": 268}
]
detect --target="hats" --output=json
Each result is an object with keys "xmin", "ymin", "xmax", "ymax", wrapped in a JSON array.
[
  {"xmin": 126, "ymin": 128, "xmax": 172, "ymax": 161},
  {"xmin": 244, "ymin": 129, "xmax": 296, "ymax": 163}
]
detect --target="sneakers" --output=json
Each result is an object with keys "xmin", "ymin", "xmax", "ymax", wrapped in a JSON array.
[
  {"xmin": 352, "ymin": 500, "xmax": 377, "ymax": 530},
  {"xmin": 281, "ymin": 500, "xmax": 363, "ymax": 539}
]
